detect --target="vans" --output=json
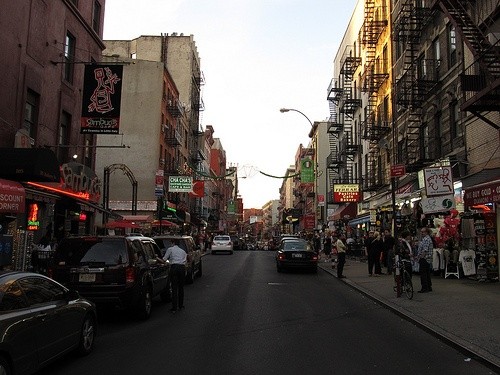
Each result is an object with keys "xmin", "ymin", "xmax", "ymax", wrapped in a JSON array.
[{"xmin": 54, "ymin": 236, "xmax": 175, "ymax": 318}]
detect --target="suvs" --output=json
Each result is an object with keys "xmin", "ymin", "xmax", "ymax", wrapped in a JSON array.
[{"xmin": 152, "ymin": 236, "xmax": 202, "ymax": 281}]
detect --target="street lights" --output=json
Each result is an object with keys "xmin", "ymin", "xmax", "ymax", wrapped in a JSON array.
[
  {"xmin": 280, "ymin": 109, "xmax": 317, "ymax": 234},
  {"xmin": 231, "ymin": 177, "xmax": 246, "ymax": 182}
]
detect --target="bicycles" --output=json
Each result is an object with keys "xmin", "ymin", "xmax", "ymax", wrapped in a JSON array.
[{"xmin": 393, "ymin": 258, "xmax": 414, "ymax": 300}]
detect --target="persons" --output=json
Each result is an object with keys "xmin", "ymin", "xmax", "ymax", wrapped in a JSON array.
[
  {"xmin": 33, "ymin": 235, "xmax": 52, "ymax": 273},
  {"xmin": 313, "ymin": 233, "xmax": 337, "ymax": 263},
  {"xmin": 336, "ymin": 232, "xmax": 348, "ymax": 278},
  {"xmin": 415, "ymin": 227, "xmax": 434, "ymax": 294},
  {"xmin": 399, "ymin": 231, "xmax": 413, "ymax": 278},
  {"xmin": 382, "ymin": 229, "xmax": 396, "ymax": 275},
  {"xmin": 203, "ymin": 233, "xmax": 214, "ymax": 250},
  {"xmin": 162, "ymin": 238, "xmax": 188, "ymax": 313},
  {"xmin": 346, "ymin": 234, "xmax": 368, "ymax": 245},
  {"xmin": 364, "ymin": 230, "xmax": 384, "ymax": 276}
]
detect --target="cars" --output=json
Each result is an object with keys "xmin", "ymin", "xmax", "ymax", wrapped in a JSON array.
[
  {"xmin": 275, "ymin": 236, "xmax": 318, "ymax": 274},
  {"xmin": 211, "ymin": 235, "xmax": 233, "ymax": 255},
  {"xmin": 0, "ymin": 271, "xmax": 97, "ymax": 375}
]
